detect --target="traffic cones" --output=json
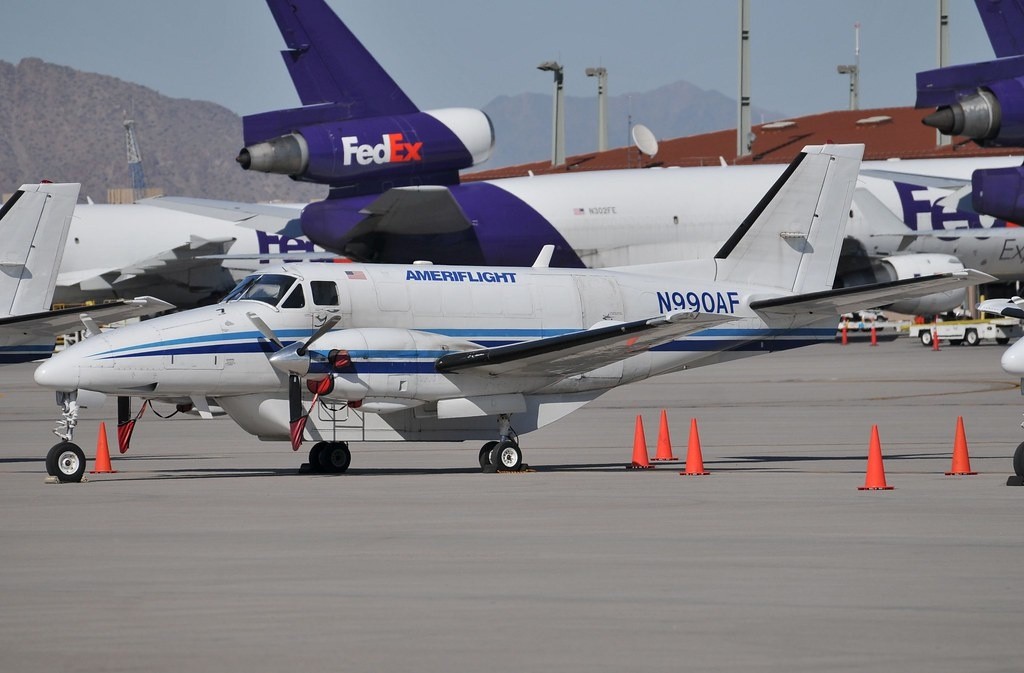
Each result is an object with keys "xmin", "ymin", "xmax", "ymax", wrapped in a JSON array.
[
  {"xmin": 857, "ymin": 424, "xmax": 895, "ymax": 491},
  {"xmin": 89, "ymin": 421, "xmax": 118, "ymax": 474},
  {"xmin": 930, "ymin": 320, "xmax": 942, "ymax": 351},
  {"xmin": 650, "ymin": 410, "xmax": 679, "ymax": 461},
  {"xmin": 868, "ymin": 316, "xmax": 879, "ymax": 347},
  {"xmin": 679, "ymin": 418, "xmax": 711, "ymax": 476},
  {"xmin": 841, "ymin": 315, "xmax": 849, "ymax": 345},
  {"xmin": 626, "ymin": 414, "xmax": 656, "ymax": 469},
  {"xmin": 945, "ymin": 416, "xmax": 978, "ymax": 476}
]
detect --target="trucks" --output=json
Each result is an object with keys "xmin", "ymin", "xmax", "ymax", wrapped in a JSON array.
[{"xmin": 910, "ymin": 304, "xmax": 1024, "ymax": 346}]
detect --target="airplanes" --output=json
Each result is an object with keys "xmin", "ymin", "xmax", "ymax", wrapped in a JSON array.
[
  {"xmin": 0, "ymin": 183, "xmax": 318, "ymax": 365},
  {"xmin": 32, "ymin": 142, "xmax": 1024, "ymax": 485},
  {"xmin": 914, "ymin": 0, "xmax": 1024, "ymax": 487}
]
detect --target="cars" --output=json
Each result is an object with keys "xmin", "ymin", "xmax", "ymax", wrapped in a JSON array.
[{"xmin": 840, "ymin": 310, "xmax": 889, "ymax": 332}]
